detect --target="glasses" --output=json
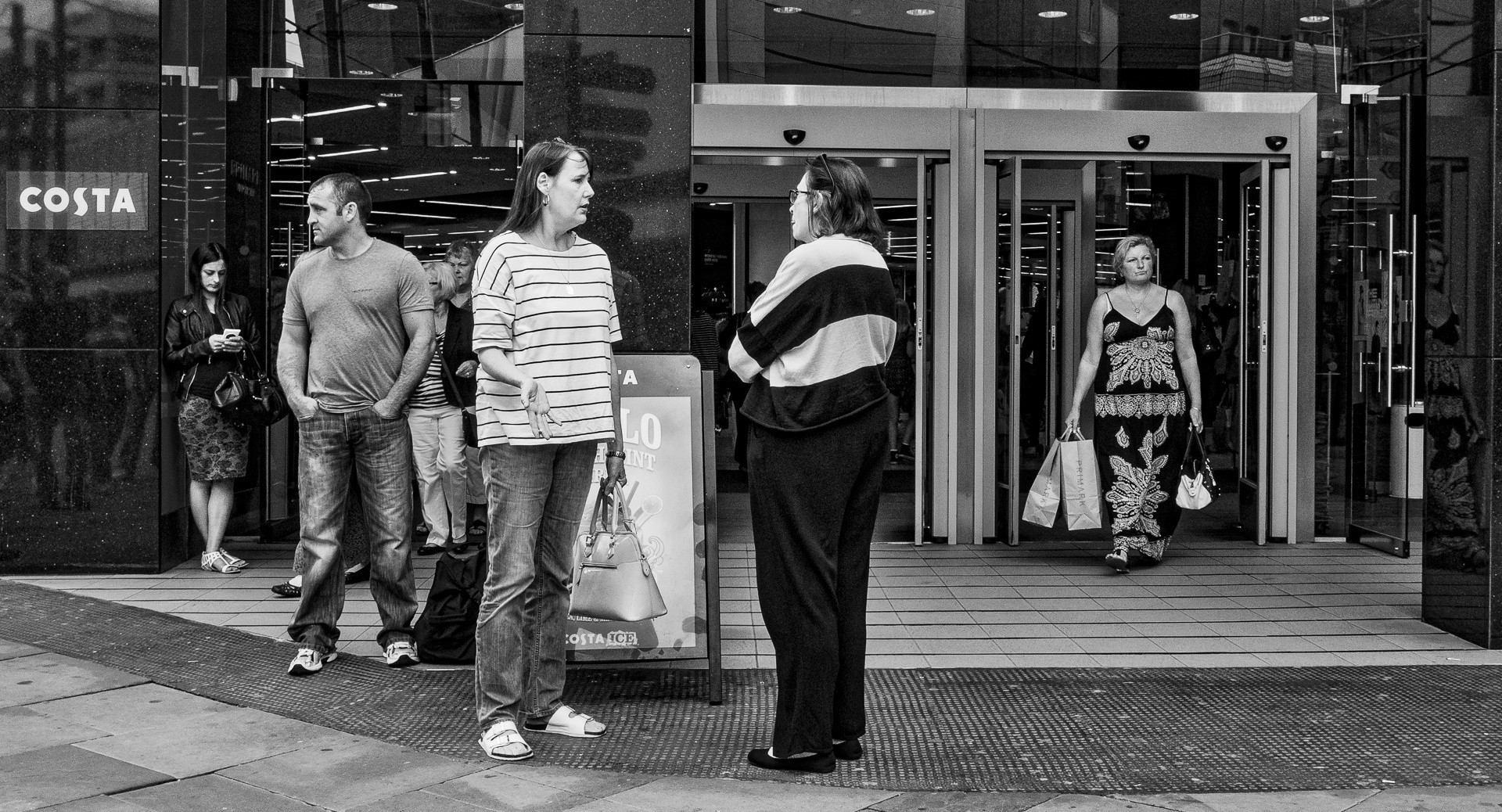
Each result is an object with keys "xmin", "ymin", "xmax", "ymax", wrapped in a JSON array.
[
  {"xmin": 789, "ymin": 189, "xmax": 814, "ymax": 205},
  {"xmin": 816, "ymin": 153, "xmax": 834, "ymax": 199},
  {"xmin": 546, "ymin": 137, "xmax": 570, "ymax": 155}
]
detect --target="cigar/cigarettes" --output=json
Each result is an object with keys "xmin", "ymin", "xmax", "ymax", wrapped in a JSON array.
[{"xmin": 539, "ymin": 429, "xmax": 542, "ymax": 435}]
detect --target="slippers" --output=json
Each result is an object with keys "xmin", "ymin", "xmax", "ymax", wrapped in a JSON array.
[
  {"xmin": 478, "ymin": 721, "xmax": 533, "ymax": 761},
  {"xmin": 523, "ymin": 705, "xmax": 607, "ymax": 738}
]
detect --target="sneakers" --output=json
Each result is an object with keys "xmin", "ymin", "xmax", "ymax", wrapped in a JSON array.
[
  {"xmin": 288, "ymin": 648, "xmax": 338, "ymax": 675},
  {"xmin": 383, "ymin": 641, "xmax": 420, "ymax": 667}
]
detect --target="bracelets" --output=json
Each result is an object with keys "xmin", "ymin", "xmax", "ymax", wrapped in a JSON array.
[{"xmin": 605, "ymin": 451, "xmax": 625, "ymax": 459}]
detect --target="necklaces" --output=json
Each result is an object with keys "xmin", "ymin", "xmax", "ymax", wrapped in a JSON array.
[
  {"xmin": 1126, "ymin": 284, "xmax": 1151, "ymax": 319},
  {"xmin": 529, "ymin": 227, "xmax": 573, "ymax": 295}
]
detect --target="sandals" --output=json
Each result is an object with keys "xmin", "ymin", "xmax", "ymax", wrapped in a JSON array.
[
  {"xmin": 1106, "ymin": 546, "xmax": 1129, "ymax": 574},
  {"xmin": 415, "ymin": 519, "xmax": 487, "ymax": 536},
  {"xmin": 201, "ymin": 551, "xmax": 241, "ymax": 573},
  {"xmin": 219, "ymin": 547, "xmax": 249, "ymax": 568},
  {"xmin": 1139, "ymin": 551, "xmax": 1157, "ymax": 564}
]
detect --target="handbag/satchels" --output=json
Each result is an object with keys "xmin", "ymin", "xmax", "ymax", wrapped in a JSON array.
[
  {"xmin": 222, "ymin": 340, "xmax": 287, "ymax": 431},
  {"xmin": 462, "ymin": 410, "xmax": 480, "ymax": 449},
  {"xmin": 1060, "ymin": 427, "xmax": 1103, "ymax": 531},
  {"xmin": 441, "ymin": 359, "xmax": 477, "ymax": 407},
  {"xmin": 1022, "ymin": 428, "xmax": 1075, "ymax": 528},
  {"xmin": 413, "ymin": 542, "xmax": 488, "ymax": 664},
  {"xmin": 1176, "ymin": 426, "xmax": 1221, "ymax": 510},
  {"xmin": 568, "ymin": 480, "xmax": 667, "ymax": 621},
  {"xmin": 212, "ymin": 340, "xmax": 248, "ymax": 408}
]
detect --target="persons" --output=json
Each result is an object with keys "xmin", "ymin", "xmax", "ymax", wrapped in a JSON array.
[
  {"xmin": 1172, "ymin": 278, "xmax": 1237, "ymax": 454},
  {"xmin": 276, "ymin": 173, "xmax": 436, "ymax": 678},
  {"xmin": 692, "ymin": 281, "xmax": 766, "ymax": 471},
  {"xmin": 887, "ymin": 298, "xmax": 915, "ymax": 464},
  {"xmin": 1066, "ymin": 234, "xmax": 1204, "ymax": 575},
  {"xmin": 725, "ymin": 154, "xmax": 897, "ymax": 774},
  {"xmin": 0, "ymin": 262, "xmax": 150, "ymax": 511},
  {"xmin": 1428, "ymin": 239, "xmax": 1495, "ymax": 574},
  {"xmin": 406, "ymin": 262, "xmax": 477, "ymax": 555},
  {"xmin": 164, "ymin": 242, "xmax": 261, "ymax": 574},
  {"xmin": 472, "ymin": 140, "xmax": 627, "ymax": 762},
  {"xmin": 415, "ymin": 239, "xmax": 489, "ymax": 538},
  {"xmin": 271, "ymin": 467, "xmax": 373, "ymax": 597},
  {"xmin": 1021, "ymin": 296, "xmax": 1062, "ymax": 450}
]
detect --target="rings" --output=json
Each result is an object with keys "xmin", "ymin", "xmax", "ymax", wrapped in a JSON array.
[
  {"xmin": 542, "ymin": 415, "xmax": 545, "ymax": 416},
  {"xmin": 466, "ymin": 372, "xmax": 470, "ymax": 376}
]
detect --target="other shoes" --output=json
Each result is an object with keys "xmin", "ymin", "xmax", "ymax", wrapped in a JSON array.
[
  {"xmin": 417, "ymin": 536, "xmax": 469, "ymax": 556},
  {"xmin": 833, "ymin": 739, "xmax": 863, "ymax": 760},
  {"xmin": 748, "ymin": 748, "xmax": 836, "ymax": 773},
  {"xmin": 272, "ymin": 581, "xmax": 301, "ymax": 597},
  {"xmin": 345, "ymin": 561, "xmax": 370, "ymax": 584}
]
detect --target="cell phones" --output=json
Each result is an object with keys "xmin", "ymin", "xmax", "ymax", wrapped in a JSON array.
[{"xmin": 222, "ymin": 329, "xmax": 241, "ymax": 339}]
